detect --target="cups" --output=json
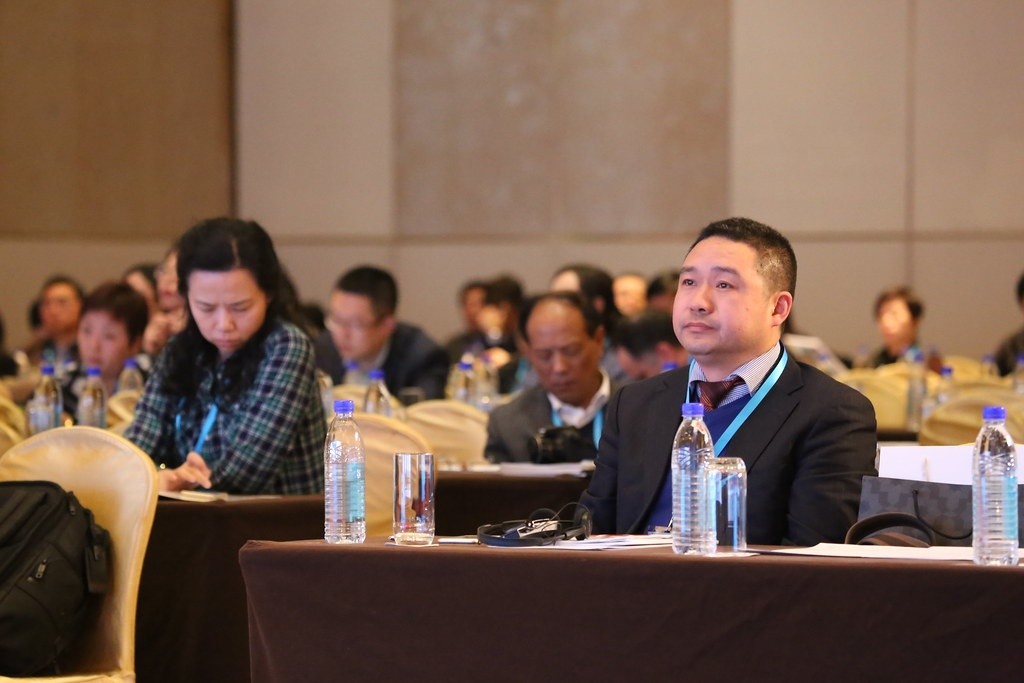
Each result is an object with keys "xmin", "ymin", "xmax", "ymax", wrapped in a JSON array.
[
  {"xmin": 25, "ymin": 400, "xmax": 50, "ymax": 438},
  {"xmin": 392, "ymin": 452, "xmax": 435, "ymax": 547},
  {"xmin": 32, "ymin": 354, "xmax": 75, "ymax": 427},
  {"xmin": 700, "ymin": 457, "xmax": 748, "ymax": 554}
]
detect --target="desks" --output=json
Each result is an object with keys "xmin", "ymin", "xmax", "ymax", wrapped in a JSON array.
[
  {"xmin": 232, "ymin": 528, "xmax": 1024, "ymax": 683},
  {"xmin": 435, "ymin": 465, "xmax": 591, "ymax": 536},
  {"xmin": 135, "ymin": 495, "xmax": 325, "ymax": 683}
]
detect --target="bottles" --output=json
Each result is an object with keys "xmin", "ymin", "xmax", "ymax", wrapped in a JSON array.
[
  {"xmin": 817, "ymin": 347, "xmax": 1024, "ymax": 434},
  {"xmin": 452, "ymin": 340, "xmax": 498, "ymax": 413},
  {"xmin": 115, "ymin": 358, "xmax": 144, "ymax": 394},
  {"xmin": 339, "ymin": 359, "xmax": 367, "ymax": 385},
  {"xmin": 970, "ymin": 405, "xmax": 1020, "ymax": 567},
  {"xmin": 323, "ymin": 399, "xmax": 367, "ymax": 544},
  {"xmin": 670, "ymin": 402, "xmax": 717, "ymax": 556},
  {"xmin": 77, "ymin": 366, "xmax": 107, "ymax": 431},
  {"xmin": 362, "ymin": 370, "xmax": 394, "ymax": 418}
]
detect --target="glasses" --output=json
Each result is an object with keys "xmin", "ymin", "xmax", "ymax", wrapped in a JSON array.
[{"xmin": 321, "ymin": 318, "xmax": 387, "ymax": 338}]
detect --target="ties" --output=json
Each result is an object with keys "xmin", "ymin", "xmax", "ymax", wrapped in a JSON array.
[{"xmin": 697, "ymin": 376, "xmax": 745, "ymax": 410}]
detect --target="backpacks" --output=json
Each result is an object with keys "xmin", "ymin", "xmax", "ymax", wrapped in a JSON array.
[{"xmin": 0, "ymin": 483, "xmax": 111, "ymax": 676}]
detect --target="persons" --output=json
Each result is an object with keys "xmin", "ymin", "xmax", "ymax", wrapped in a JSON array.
[
  {"xmin": 0, "ymin": 240, "xmax": 188, "ymax": 437},
  {"xmin": 124, "ymin": 216, "xmax": 327, "ymax": 496},
  {"xmin": 864, "ymin": 286, "xmax": 925, "ymax": 368},
  {"xmin": 587, "ymin": 217, "xmax": 877, "ymax": 548},
  {"xmin": 292, "ymin": 259, "xmax": 692, "ymax": 468},
  {"xmin": 995, "ymin": 273, "xmax": 1024, "ymax": 377}
]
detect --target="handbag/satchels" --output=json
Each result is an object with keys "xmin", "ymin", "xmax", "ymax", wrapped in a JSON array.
[{"xmin": 858, "ymin": 476, "xmax": 1024, "ymax": 547}]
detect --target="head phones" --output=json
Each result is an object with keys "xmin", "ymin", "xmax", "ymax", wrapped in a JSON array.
[
  {"xmin": 527, "ymin": 425, "xmax": 588, "ymax": 464},
  {"xmin": 476, "ymin": 508, "xmax": 593, "ymax": 547}
]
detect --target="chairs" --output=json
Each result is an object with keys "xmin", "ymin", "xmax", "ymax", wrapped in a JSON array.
[{"xmin": 0, "ymin": 345, "xmax": 1024, "ymax": 683}]
